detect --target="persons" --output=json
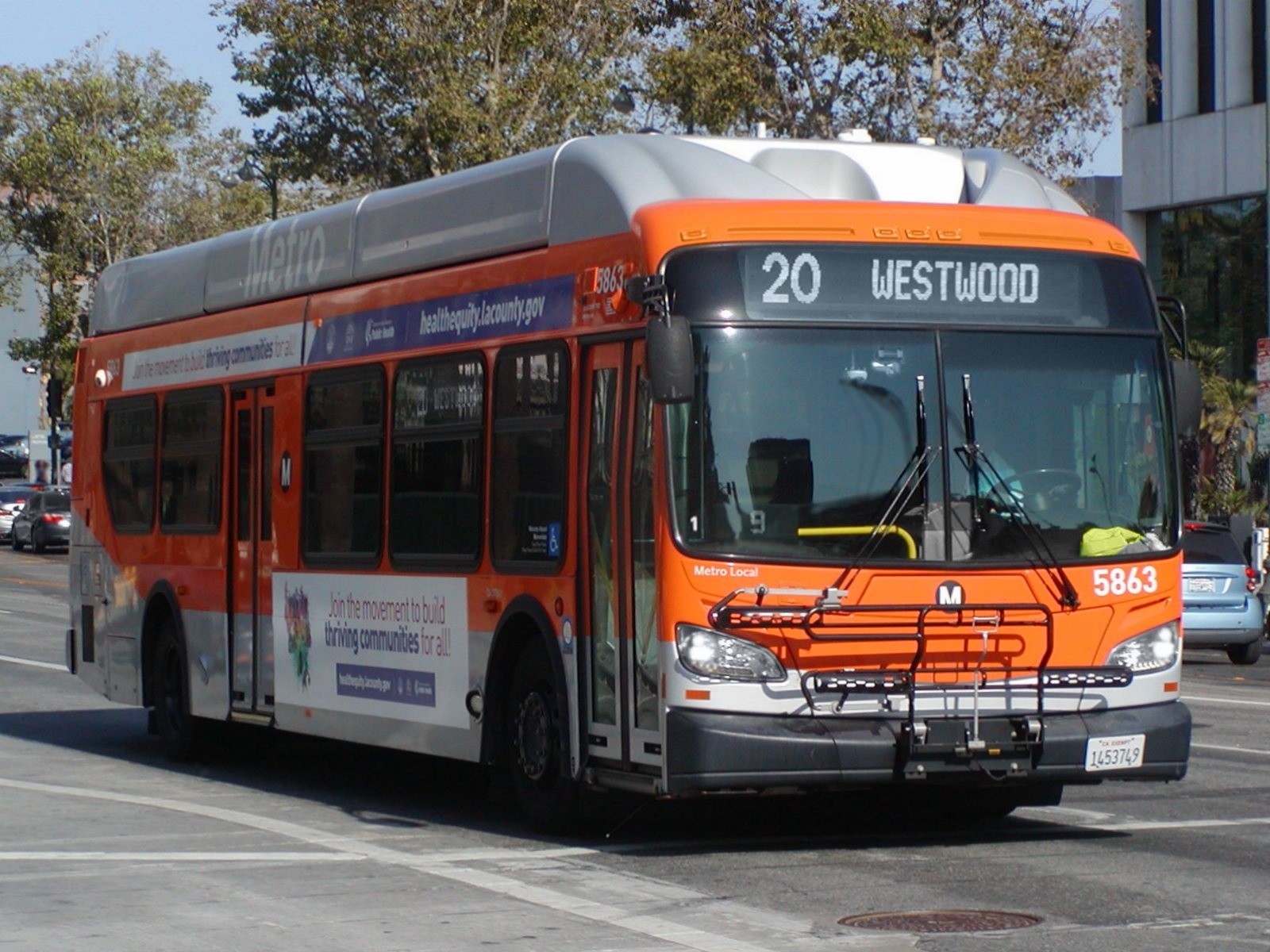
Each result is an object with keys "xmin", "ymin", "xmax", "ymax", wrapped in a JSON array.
[{"xmin": 61, "ymin": 461, "xmax": 73, "ymax": 485}]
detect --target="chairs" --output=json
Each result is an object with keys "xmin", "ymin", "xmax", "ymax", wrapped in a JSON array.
[
  {"xmin": 108, "ymin": 505, "xmax": 150, "ymax": 534},
  {"xmin": 164, "ymin": 494, "xmax": 250, "ymax": 540}
]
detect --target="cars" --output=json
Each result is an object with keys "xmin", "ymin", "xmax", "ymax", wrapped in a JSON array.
[
  {"xmin": 1140, "ymin": 520, "xmax": 1270, "ymax": 666},
  {"xmin": 10, "ymin": 488, "xmax": 71, "ymax": 555},
  {"xmin": 0, "ymin": 420, "xmax": 74, "ymax": 546}
]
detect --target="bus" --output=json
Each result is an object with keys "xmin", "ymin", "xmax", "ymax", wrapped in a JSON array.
[{"xmin": 63, "ymin": 128, "xmax": 1204, "ymax": 840}]
